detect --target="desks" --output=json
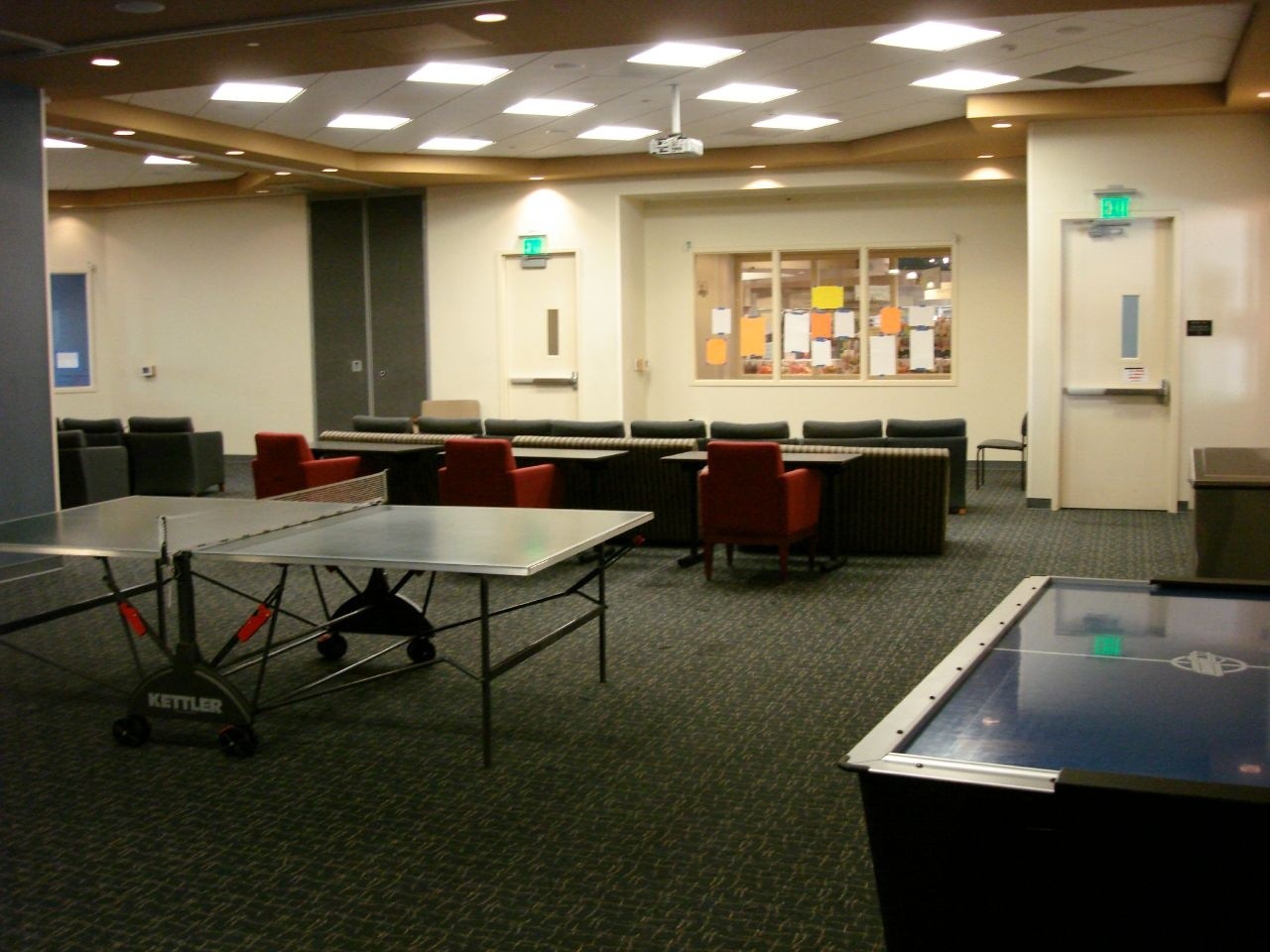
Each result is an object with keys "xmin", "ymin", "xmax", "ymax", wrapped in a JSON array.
[
  {"xmin": 664, "ymin": 450, "xmax": 859, "ymax": 577},
  {"xmin": 512, "ymin": 449, "xmax": 627, "ymax": 513},
  {"xmin": 309, "ymin": 441, "xmax": 440, "ymax": 505},
  {"xmin": 0, "ymin": 492, "xmax": 657, "ymax": 777}
]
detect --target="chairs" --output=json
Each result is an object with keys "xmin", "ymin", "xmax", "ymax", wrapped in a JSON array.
[
  {"xmin": 253, "ymin": 430, "xmax": 361, "ymax": 503},
  {"xmin": 57, "ymin": 417, "xmax": 224, "ymax": 507},
  {"xmin": 976, "ymin": 415, "xmax": 1029, "ymax": 489},
  {"xmin": 354, "ymin": 416, "xmax": 968, "ymax": 510},
  {"xmin": 693, "ymin": 441, "xmax": 821, "ymax": 583}
]
